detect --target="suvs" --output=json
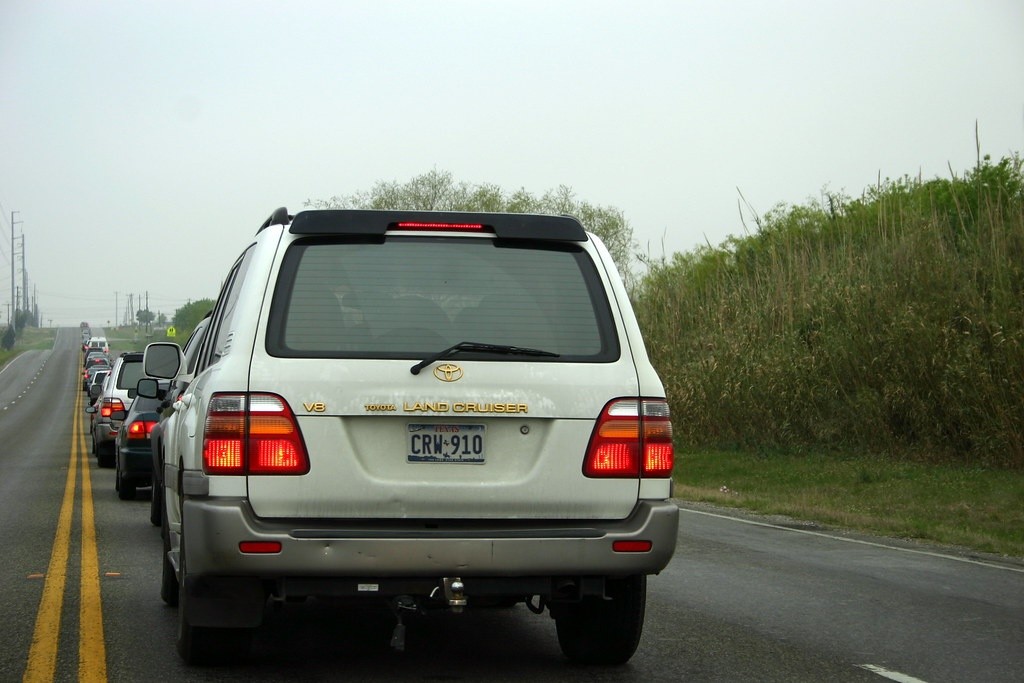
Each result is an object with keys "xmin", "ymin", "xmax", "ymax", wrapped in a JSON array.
[{"xmin": 143, "ymin": 205, "xmax": 681, "ymax": 669}]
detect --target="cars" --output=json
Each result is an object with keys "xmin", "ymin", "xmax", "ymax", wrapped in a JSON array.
[{"xmin": 79, "ymin": 322, "xmax": 219, "ymax": 526}]
